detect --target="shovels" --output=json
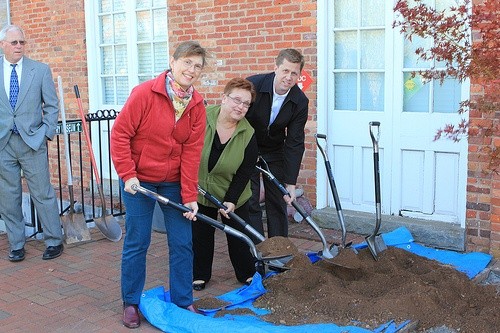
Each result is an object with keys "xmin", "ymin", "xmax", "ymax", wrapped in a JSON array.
[
  {"xmin": 254, "ymin": 155, "xmax": 363, "ymax": 270},
  {"xmin": 196, "ymin": 181, "xmax": 295, "ymax": 270},
  {"xmin": 129, "ymin": 183, "xmax": 294, "ymax": 260},
  {"xmin": 56, "ymin": 74, "xmax": 92, "ymax": 245},
  {"xmin": 73, "ymin": 83, "xmax": 123, "ymax": 242},
  {"xmin": 313, "ymin": 132, "xmax": 359, "ymax": 261},
  {"xmin": 364, "ymin": 120, "xmax": 387, "ymax": 261}
]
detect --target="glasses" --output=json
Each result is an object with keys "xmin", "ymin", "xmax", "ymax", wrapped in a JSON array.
[
  {"xmin": 227, "ymin": 94, "xmax": 251, "ymax": 108},
  {"xmin": 2, "ymin": 39, "xmax": 25, "ymax": 45},
  {"xmin": 177, "ymin": 55, "xmax": 203, "ymax": 72}
]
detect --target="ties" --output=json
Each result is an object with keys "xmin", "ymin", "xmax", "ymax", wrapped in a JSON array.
[{"xmin": 10, "ymin": 64, "xmax": 19, "ymax": 134}]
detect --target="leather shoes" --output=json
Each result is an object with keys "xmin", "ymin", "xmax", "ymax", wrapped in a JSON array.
[
  {"xmin": 9, "ymin": 247, "xmax": 25, "ymax": 260},
  {"xmin": 43, "ymin": 243, "xmax": 64, "ymax": 259}
]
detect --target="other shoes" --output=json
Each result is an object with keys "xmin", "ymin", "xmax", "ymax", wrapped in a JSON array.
[
  {"xmin": 240, "ymin": 278, "xmax": 252, "ymax": 286},
  {"xmin": 123, "ymin": 302, "xmax": 140, "ymax": 328},
  {"xmin": 255, "ymin": 261, "xmax": 263, "ymax": 266},
  {"xmin": 192, "ymin": 280, "xmax": 205, "ymax": 291},
  {"xmin": 181, "ymin": 304, "xmax": 199, "ymax": 313}
]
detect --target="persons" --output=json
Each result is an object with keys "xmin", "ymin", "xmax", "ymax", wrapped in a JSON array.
[
  {"xmin": 191, "ymin": 79, "xmax": 259, "ymax": 290},
  {"xmin": 0, "ymin": 25, "xmax": 65, "ymax": 262},
  {"xmin": 244, "ymin": 49, "xmax": 309, "ymax": 239},
  {"xmin": 110, "ymin": 41, "xmax": 206, "ymax": 329}
]
